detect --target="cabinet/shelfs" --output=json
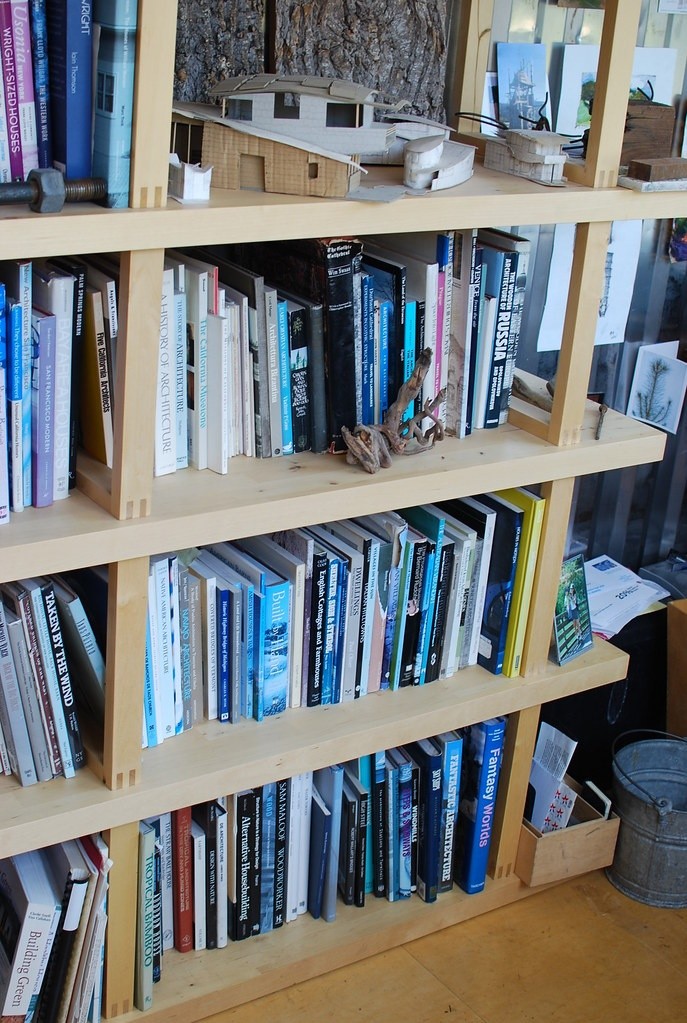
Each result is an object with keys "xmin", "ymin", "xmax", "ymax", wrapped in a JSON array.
[{"xmin": 0, "ymin": 0, "xmax": 687, "ymax": 1023}]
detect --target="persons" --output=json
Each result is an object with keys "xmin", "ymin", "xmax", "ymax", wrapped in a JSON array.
[{"xmin": 563, "ymin": 584, "xmax": 582, "ymax": 640}]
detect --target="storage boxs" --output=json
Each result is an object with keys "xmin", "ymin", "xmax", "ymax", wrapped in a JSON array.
[{"xmin": 512, "ymin": 768, "xmax": 621, "ymax": 890}]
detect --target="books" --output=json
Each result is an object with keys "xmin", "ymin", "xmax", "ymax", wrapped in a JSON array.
[
  {"xmin": 0, "ymin": 717, "xmax": 506, "ymax": 1023},
  {"xmin": 0, "ymin": 0, "xmax": 138, "ymax": 209},
  {"xmin": 581, "ymin": 780, "xmax": 615, "ymax": 820},
  {"xmin": 0, "ymin": 227, "xmax": 531, "ymax": 525},
  {"xmin": 0, "ymin": 486, "xmax": 546, "ymax": 787}
]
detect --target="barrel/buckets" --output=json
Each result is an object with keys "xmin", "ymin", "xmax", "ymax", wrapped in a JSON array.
[{"xmin": 605, "ymin": 729, "xmax": 687, "ymax": 909}]
